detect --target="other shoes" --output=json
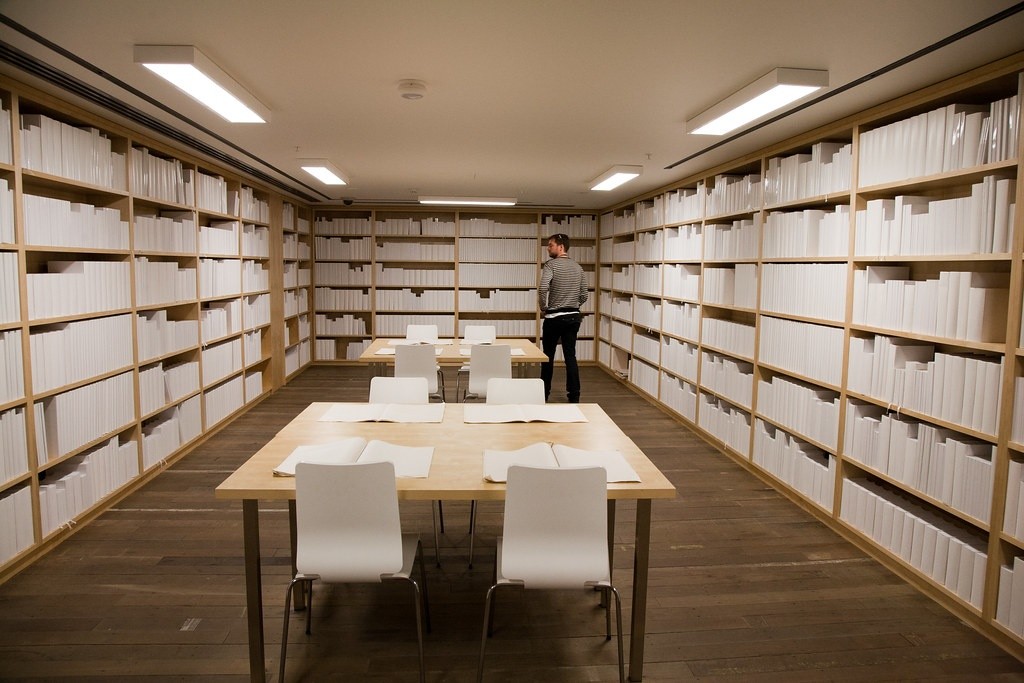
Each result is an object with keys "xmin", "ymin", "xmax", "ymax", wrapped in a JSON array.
[{"xmin": 567, "ymin": 393, "xmax": 580, "ymax": 403}]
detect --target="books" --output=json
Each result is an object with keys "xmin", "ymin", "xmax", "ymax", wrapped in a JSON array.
[{"xmin": 0, "ymin": 94, "xmax": 1024, "ymax": 640}]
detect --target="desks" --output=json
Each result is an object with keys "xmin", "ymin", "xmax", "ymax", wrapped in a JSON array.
[
  {"xmin": 359, "ymin": 338, "xmax": 548, "ymax": 400},
  {"xmin": 214, "ymin": 403, "xmax": 677, "ymax": 683}
]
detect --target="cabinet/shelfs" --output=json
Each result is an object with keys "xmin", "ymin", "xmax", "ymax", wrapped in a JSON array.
[{"xmin": 0, "ymin": 55, "xmax": 1024, "ymax": 667}]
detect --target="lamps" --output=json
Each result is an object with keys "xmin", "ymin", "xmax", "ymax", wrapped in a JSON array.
[
  {"xmin": 418, "ymin": 196, "xmax": 517, "ymax": 204},
  {"xmin": 588, "ymin": 165, "xmax": 642, "ymax": 192},
  {"xmin": 132, "ymin": 45, "xmax": 271, "ymax": 125},
  {"xmin": 296, "ymin": 157, "xmax": 350, "ymax": 186},
  {"xmin": 686, "ymin": 69, "xmax": 828, "ymax": 137}
]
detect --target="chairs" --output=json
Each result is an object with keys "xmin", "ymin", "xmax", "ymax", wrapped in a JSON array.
[
  {"xmin": 462, "ymin": 345, "xmax": 510, "ymax": 404},
  {"xmin": 369, "ymin": 377, "xmax": 444, "ymax": 568},
  {"xmin": 406, "ymin": 325, "xmax": 445, "ymax": 401},
  {"xmin": 454, "ymin": 378, "xmax": 546, "ymax": 566},
  {"xmin": 476, "ymin": 465, "xmax": 625, "ymax": 683},
  {"xmin": 277, "ymin": 459, "xmax": 429, "ymax": 682},
  {"xmin": 456, "ymin": 326, "xmax": 496, "ymax": 401},
  {"xmin": 395, "ymin": 345, "xmax": 445, "ymax": 403}
]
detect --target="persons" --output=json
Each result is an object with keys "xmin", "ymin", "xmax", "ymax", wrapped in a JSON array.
[{"xmin": 537, "ymin": 233, "xmax": 589, "ymax": 403}]
